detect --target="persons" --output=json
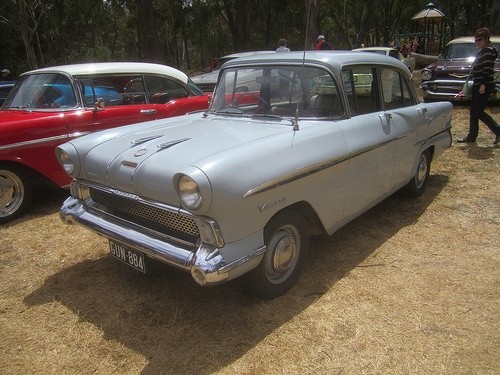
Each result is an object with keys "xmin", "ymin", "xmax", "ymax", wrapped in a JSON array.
[
  {"xmin": 276, "ymin": 38, "xmax": 290, "ymax": 88},
  {"xmin": 457, "ymin": 26, "xmax": 500, "ymax": 145},
  {"xmin": 316, "ymin": 35, "xmax": 334, "ymax": 49}
]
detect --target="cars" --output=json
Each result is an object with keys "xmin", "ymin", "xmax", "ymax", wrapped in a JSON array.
[
  {"xmin": 311, "ymin": 48, "xmax": 418, "ymax": 96},
  {"xmin": 0, "ymin": 79, "xmax": 123, "ymax": 110},
  {"xmin": 102, "ymin": 74, "xmax": 172, "ymax": 106},
  {"xmin": 421, "ymin": 36, "xmax": 500, "ymax": 101},
  {"xmin": 51, "ymin": 47, "xmax": 461, "ymax": 303},
  {"xmin": 187, "ymin": 51, "xmax": 303, "ymax": 92},
  {"xmin": 0, "ymin": 63, "xmax": 263, "ymax": 225}
]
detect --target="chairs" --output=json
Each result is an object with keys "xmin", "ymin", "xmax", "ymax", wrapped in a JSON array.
[
  {"xmin": 309, "ymin": 95, "xmax": 376, "ymax": 115},
  {"xmin": 123, "ymin": 95, "xmax": 142, "ymax": 104},
  {"xmin": 150, "ymin": 93, "xmax": 169, "ymax": 104}
]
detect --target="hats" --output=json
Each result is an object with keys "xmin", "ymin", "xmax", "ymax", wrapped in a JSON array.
[
  {"xmin": 475, "ymin": 28, "xmax": 490, "ymax": 40},
  {"xmin": 318, "ymin": 35, "xmax": 325, "ymax": 40}
]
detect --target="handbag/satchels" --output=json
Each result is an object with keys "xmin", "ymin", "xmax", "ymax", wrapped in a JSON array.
[{"xmin": 462, "ymin": 82, "xmax": 473, "ymax": 95}]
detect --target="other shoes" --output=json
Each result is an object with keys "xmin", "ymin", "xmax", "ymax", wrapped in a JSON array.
[
  {"xmin": 458, "ymin": 135, "xmax": 476, "ymax": 143},
  {"xmin": 493, "ymin": 136, "xmax": 500, "ymax": 144}
]
